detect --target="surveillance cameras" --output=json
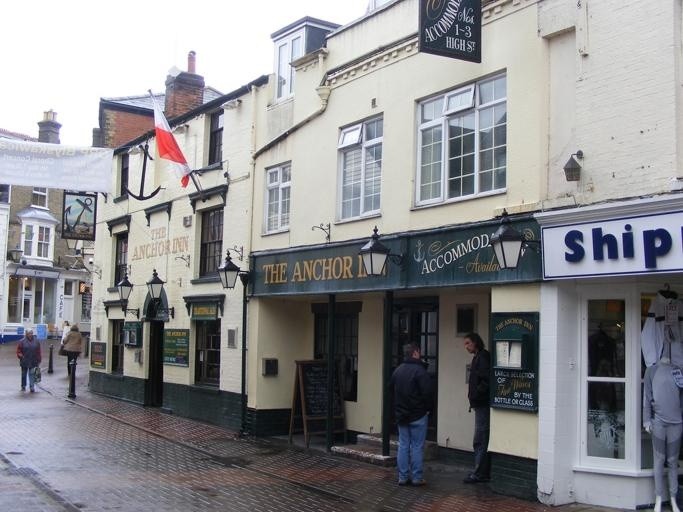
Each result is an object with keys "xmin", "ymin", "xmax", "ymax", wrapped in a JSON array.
[
  {"xmin": 89, "ymin": 258, "xmax": 94, "ymax": 264},
  {"xmin": 224, "ymin": 169, "xmax": 232, "ymax": 178}
]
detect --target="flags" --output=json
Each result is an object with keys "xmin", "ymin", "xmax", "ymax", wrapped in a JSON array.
[{"xmin": 152, "ymin": 97, "xmax": 193, "ymax": 189}]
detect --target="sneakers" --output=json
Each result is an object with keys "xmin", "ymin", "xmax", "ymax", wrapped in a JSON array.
[
  {"xmin": 464, "ymin": 477, "xmax": 480, "ymax": 483},
  {"xmin": 21, "ymin": 387, "xmax": 34, "ymax": 392},
  {"xmin": 399, "ymin": 479, "xmax": 426, "ymax": 486}
]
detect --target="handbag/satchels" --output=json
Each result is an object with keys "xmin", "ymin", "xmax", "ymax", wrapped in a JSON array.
[
  {"xmin": 59, "ymin": 345, "xmax": 67, "ymax": 355},
  {"xmin": 34, "ymin": 367, "xmax": 41, "ymax": 383}
]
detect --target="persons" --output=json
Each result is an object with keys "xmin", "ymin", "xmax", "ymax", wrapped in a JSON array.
[
  {"xmin": 642, "ymin": 356, "xmax": 683, "ymax": 511},
  {"xmin": 591, "ymin": 358, "xmax": 619, "ymax": 458},
  {"xmin": 16, "ymin": 326, "xmax": 42, "ymax": 393},
  {"xmin": 461, "ymin": 332, "xmax": 491, "ymax": 483},
  {"xmin": 62, "ymin": 325, "xmax": 82, "ymax": 376},
  {"xmin": 59, "ymin": 320, "xmax": 70, "ymax": 346},
  {"xmin": 388, "ymin": 344, "xmax": 432, "ymax": 485}
]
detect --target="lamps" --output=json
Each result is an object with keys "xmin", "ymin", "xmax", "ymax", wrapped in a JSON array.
[
  {"xmin": 9, "ymin": 245, "xmax": 24, "ymax": 263},
  {"xmin": 359, "ymin": 225, "xmax": 402, "ymax": 275},
  {"xmin": 563, "ymin": 150, "xmax": 583, "ymax": 181},
  {"xmin": 79, "ymin": 280, "xmax": 87, "ymax": 295},
  {"xmin": 489, "ymin": 208, "xmax": 542, "ymax": 268},
  {"xmin": 145, "ymin": 267, "xmax": 176, "ymax": 318},
  {"xmin": 218, "ymin": 251, "xmax": 250, "ymax": 289},
  {"xmin": 118, "ymin": 273, "xmax": 139, "ymax": 319}
]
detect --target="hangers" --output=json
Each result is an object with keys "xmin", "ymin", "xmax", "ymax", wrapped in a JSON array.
[{"xmin": 659, "ymin": 283, "xmax": 681, "ymax": 302}]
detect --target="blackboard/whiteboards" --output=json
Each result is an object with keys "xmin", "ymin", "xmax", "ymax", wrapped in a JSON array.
[
  {"xmin": 299, "ymin": 360, "xmax": 344, "ymax": 420},
  {"xmin": 490, "ymin": 366, "xmax": 538, "ymax": 413}
]
